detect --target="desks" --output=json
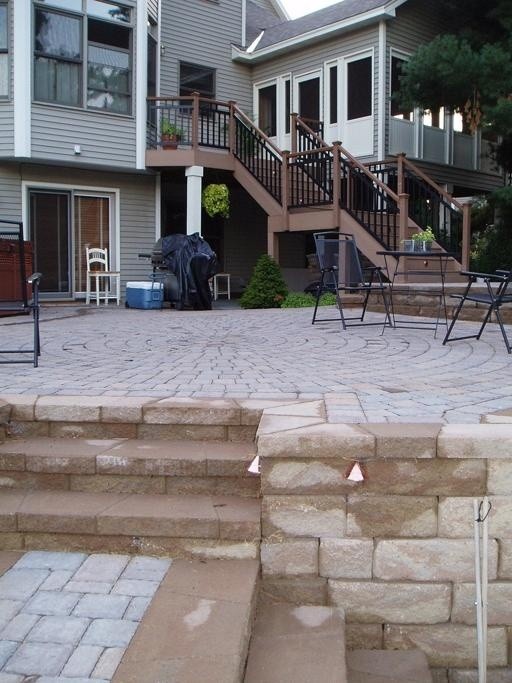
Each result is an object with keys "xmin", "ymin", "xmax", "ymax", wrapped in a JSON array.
[{"xmin": 375, "ymin": 251, "xmax": 460, "ymax": 338}]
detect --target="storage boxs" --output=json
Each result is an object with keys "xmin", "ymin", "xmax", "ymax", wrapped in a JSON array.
[{"xmin": 126, "ymin": 282, "xmax": 164, "ymax": 309}]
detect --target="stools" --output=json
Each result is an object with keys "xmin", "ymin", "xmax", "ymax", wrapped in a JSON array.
[{"xmin": 214, "ymin": 274, "xmax": 231, "ymax": 300}]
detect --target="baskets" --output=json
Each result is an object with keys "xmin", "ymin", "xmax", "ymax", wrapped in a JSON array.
[{"xmin": 403, "ymin": 240, "xmax": 432, "ymax": 252}]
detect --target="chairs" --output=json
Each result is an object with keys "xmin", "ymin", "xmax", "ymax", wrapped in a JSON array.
[
  {"xmin": 0, "ymin": 219, "xmax": 42, "ymax": 367},
  {"xmin": 305, "ymin": 232, "xmax": 391, "ymax": 330},
  {"xmin": 442, "ymin": 269, "xmax": 512, "ymax": 354},
  {"xmin": 86, "ymin": 248, "xmax": 121, "ymax": 306}
]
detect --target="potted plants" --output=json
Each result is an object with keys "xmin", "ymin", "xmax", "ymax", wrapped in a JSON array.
[
  {"xmin": 160, "ymin": 116, "xmax": 184, "ymax": 150},
  {"xmin": 412, "ymin": 226, "xmax": 436, "ymax": 251}
]
